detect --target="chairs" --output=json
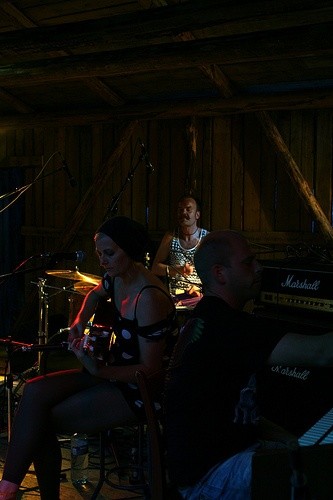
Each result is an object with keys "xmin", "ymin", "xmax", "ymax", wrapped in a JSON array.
[{"xmin": 136, "ymin": 370, "xmax": 180, "ymax": 500}]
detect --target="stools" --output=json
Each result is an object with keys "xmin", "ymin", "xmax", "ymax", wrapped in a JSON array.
[{"xmin": 80, "ymin": 417, "xmax": 151, "ymax": 500}]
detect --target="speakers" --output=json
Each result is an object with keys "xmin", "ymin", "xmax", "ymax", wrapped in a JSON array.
[{"xmin": 248, "ymin": 312, "xmax": 333, "ymax": 435}]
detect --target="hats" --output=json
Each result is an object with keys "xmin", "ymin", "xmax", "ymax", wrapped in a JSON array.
[{"xmin": 99, "ymin": 216, "xmax": 151, "ymax": 260}]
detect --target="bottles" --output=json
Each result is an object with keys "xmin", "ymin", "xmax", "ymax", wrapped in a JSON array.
[{"xmin": 70, "ymin": 433, "xmax": 89, "ymax": 486}]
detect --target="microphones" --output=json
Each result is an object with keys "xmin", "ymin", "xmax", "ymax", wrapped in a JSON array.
[
  {"xmin": 140, "ymin": 143, "xmax": 159, "ymax": 176},
  {"xmin": 42, "ymin": 250, "xmax": 86, "ymax": 262},
  {"xmin": 20, "ymin": 343, "xmax": 69, "ymax": 352},
  {"xmin": 58, "ymin": 151, "xmax": 77, "ymax": 188}
]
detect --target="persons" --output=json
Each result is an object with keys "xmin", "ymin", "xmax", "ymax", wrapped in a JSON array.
[
  {"xmin": 162, "ymin": 231, "xmax": 333, "ymax": 483},
  {"xmin": 152, "ymin": 195, "xmax": 210, "ymax": 297},
  {"xmin": 0, "ymin": 215, "xmax": 179, "ymax": 500}
]
